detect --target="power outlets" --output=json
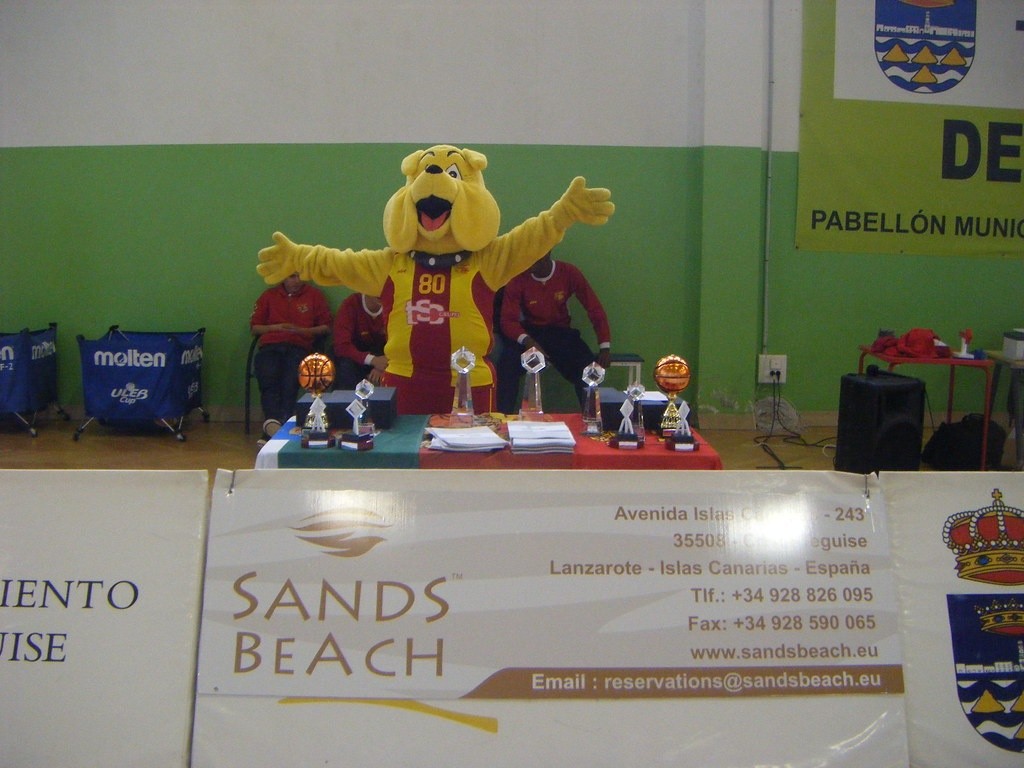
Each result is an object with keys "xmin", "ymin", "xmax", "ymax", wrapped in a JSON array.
[{"xmin": 759, "ymin": 355, "xmax": 786, "ymax": 383}]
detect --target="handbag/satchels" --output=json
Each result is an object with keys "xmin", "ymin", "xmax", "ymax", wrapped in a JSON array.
[{"xmin": 922, "ymin": 413, "xmax": 1006, "ymax": 470}]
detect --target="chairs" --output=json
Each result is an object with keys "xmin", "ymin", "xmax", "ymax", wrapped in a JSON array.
[{"xmin": 246, "ymin": 336, "xmax": 325, "ymax": 432}]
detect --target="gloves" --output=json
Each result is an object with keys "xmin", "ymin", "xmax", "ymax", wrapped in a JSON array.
[
  {"xmin": 522, "ymin": 336, "xmax": 550, "ymax": 359},
  {"xmin": 598, "ymin": 348, "xmax": 611, "ymax": 369}
]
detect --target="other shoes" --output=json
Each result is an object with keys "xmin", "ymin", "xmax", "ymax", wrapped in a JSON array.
[
  {"xmin": 264, "ymin": 419, "xmax": 282, "ymax": 438},
  {"xmin": 256, "ymin": 435, "xmax": 271, "ymax": 450}
]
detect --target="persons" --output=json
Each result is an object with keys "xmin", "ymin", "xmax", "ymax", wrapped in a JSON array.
[
  {"xmin": 250, "ymin": 275, "xmax": 332, "ymax": 449},
  {"xmin": 496, "ymin": 249, "xmax": 611, "ymax": 413},
  {"xmin": 330, "ymin": 292, "xmax": 388, "ymax": 390}
]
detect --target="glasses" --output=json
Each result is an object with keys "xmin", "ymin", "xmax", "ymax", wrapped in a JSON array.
[{"xmin": 290, "ymin": 274, "xmax": 299, "ymax": 278}]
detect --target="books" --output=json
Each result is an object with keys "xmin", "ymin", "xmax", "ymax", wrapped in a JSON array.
[
  {"xmin": 507, "ymin": 420, "xmax": 575, "ymax": 454},
  {"xmin": 426, "ymin": 427, "xmax": 508, "ymax": 452}
]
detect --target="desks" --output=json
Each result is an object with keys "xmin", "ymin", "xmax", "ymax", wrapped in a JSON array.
[
  {"xmin": 984, "ymin": 349, "xmax": 1024, "ymax": 471},
  {"xmin": 255, "ymin": 414, "xmax": 723, "ymax": 470},
  {"xmin": 859, "ymin": 345, "xmax": 996, "ymax": 472}
]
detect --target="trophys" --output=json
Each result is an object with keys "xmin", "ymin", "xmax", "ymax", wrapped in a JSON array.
[
  {"xmin": 356, "ymin": 379, "xmax": 378, "ymax": 435},
  {"xmin": 520, "ymin": 347, "xmax": 552, "ymax": 421},
  {"xmin": 654, "ymin": 354, "xmax": 690, "ymax": 438},
  {"xmin": 582, "ymin": 362, "xmax": 607, "ymax": 435},
  {"xmin": 609, "ymin": 399, "xmax": 644, "ymax": 448},
  {"xmin": 301, "ymin": 397, "xmax": 335, "ymax": 449},
  {"xmin": 666, "ymin": 401, "xmax": 699, "ymax": 451},
  {"xmin": 429, "ymin": 346, "xmax": 481, "ymax": 429},
  {"xmin": 338, "ymin": 399, "xmax": 373, "ymax": 450},
  {"xmin": 624, "ymin": 381, "xmax": 646, "ymax": 434},
  {"xmin": 298, "ymin": 353, "xmax": 336, "ymax": 430}
]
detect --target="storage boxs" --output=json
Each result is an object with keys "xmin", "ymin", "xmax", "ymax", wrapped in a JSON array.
[
  {"xmin": 297, "ymin": 387, "xmax": 397, "ymax": 429},
  {"xmin": 584, "ymin": 386, "xmax": 689, "ymax": 427},
  {"xmin": 1002, "ymin": 331, "xmax": 1024, "ymax": 361}
]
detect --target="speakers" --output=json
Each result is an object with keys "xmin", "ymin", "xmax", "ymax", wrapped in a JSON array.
[{"xmin": 834, "ymin": 372, "xmax": 925, "ymax": 474}]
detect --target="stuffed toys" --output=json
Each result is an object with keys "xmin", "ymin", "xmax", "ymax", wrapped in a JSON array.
[{"xmin": 257, "ymin": 145, "xmax": 615, "ymax": 417}]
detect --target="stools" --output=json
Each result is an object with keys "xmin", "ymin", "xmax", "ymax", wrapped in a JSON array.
[{"xmin": 595, "ymin": 353, "xmax": 644, "ymax": 388}]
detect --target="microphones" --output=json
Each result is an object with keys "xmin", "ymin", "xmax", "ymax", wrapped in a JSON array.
[{"xmin": 866, "ymin": 365, "xmax": 905, "ymax": 378}]
